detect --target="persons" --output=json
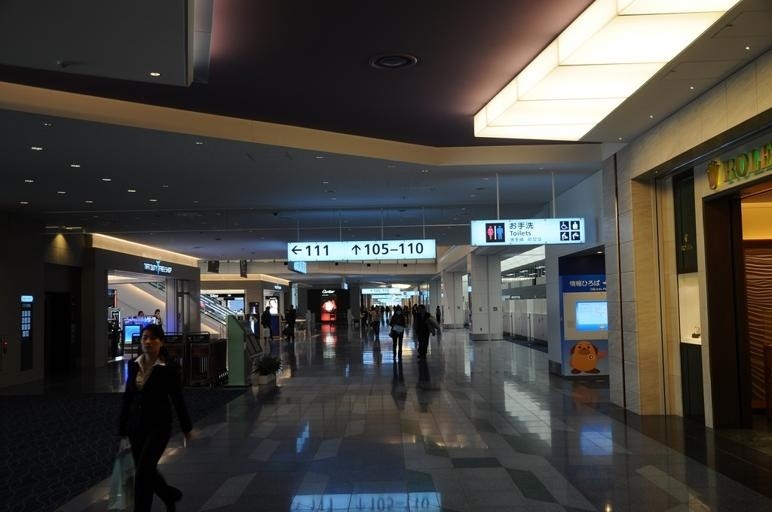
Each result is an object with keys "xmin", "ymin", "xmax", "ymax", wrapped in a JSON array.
[
  {"xmin": 413, "ymin": 305, "xmax": 441, "ymax": 359},
  {"xmin": 119, "ymin": 324, "xmax": 191, "ymax": 512},
  {"xmin": 390, "ymin": 306, "xmax": 405, "ymax": 358},
  {"xmin": 368, "ymin": 304, "xmax": 417, "ymax": 340},
  {"xmin": 153, "ymin": 309, "xmax": 161, "ymax": 323},
  {"xmin": 416, "ymin": 359, "xmax": 442, "ymax": 412},
  {"xmin": 391, "ymin": 359, "xmax": 407, "ymax": 411},
  {"xmin": 373, "ymin": 338, "xmax": 382, "ymax": 369},
  {"xmin": 261, "ymin": 307, "xmax": 273, "ymax": 339},
  {"xmin": 138, "ymin": 311, "xmax": 144, "ymax": 317},
  {"xmin": 436, "ymin": 327, "xmax": 441, "ymax": 343},
  {"xmin": 287, "ymin": 342, "xmax": 296, "ymax": 376},
  {"xmin": 436, "ymin": 306, "xmax": 441, "ymax": 324},
  {"xmin": 285, "ymin": 305, "xmax": 295, "ymax": 343}
]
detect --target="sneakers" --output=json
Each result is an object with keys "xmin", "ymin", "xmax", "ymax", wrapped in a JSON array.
[
  {"xmin": 392, "ymin": 354, "xmax": 402, "ymax": 360},
  {"xmin": 416, "ymin": 347, "xmax": 428, "ymax": 359},
  {"xmin": 164, "ymin": 487, "xmax": 183, "ymax": 511}
]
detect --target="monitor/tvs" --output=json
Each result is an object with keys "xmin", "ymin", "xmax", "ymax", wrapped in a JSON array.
[{"xmin": 563, "ymin": 292, "xmax": 608, "ymax": 340}]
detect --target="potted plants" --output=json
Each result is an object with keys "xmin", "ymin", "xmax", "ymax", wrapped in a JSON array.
[{"xmin": 253, "ymin": 355, "xmax": 283, "ymax": 384}]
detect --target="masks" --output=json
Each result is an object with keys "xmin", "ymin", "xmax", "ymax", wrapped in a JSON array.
[{"xmin": 395, "ymin": 311, "xmax": 400, "ymax": 314}]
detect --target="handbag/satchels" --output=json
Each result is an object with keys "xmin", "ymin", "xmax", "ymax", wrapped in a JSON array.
[
  {"xmin": 389, "ymin": 325, "xmax": 404, "ymax": 339},
  {"xmin": 107, "ymin": 438, "xmax": 136, "ymax": 511},
  {"xmin": 263, "ymin": 327, "xmax": 271, "ymax": 338}
]
